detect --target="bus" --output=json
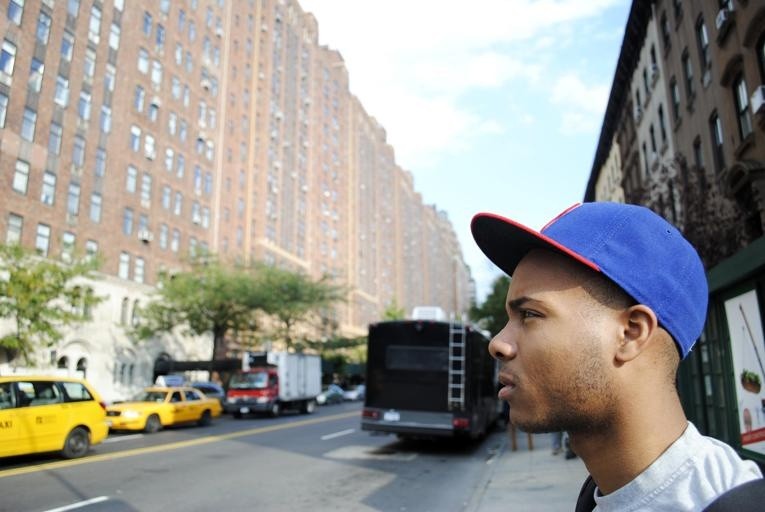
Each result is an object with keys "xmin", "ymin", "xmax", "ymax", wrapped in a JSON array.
[{"xmin": 358, "ymin": 305, "xmax": 502, "ymax": 447}]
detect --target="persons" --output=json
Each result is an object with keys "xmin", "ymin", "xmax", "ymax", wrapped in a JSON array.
[
  {"xmin": 551, "ymin": 430, "xmax": 577, "ymax": 460},
  {"xmin": 470, "ymin": 200, "xmax": 765, "ymax": 512}
]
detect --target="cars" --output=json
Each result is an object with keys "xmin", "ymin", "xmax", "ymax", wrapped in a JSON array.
[{"xmin": 107, "ymin": 381, "xmax": 225, "ymax": 431}]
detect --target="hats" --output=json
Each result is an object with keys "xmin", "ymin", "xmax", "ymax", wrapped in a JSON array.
[{"xmin": 471, "ymin": 201, "xmax": 710, "ymax": 362}]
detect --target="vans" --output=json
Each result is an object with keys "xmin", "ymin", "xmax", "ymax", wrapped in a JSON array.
[{"xmin": 1, "ymin": 372, "xmax": 109, "ymax": 460}]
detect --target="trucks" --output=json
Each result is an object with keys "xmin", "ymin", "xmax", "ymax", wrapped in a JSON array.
[{"xmin": 227, "ymin": 351, "xmax": 323, "ymax": 418}]
